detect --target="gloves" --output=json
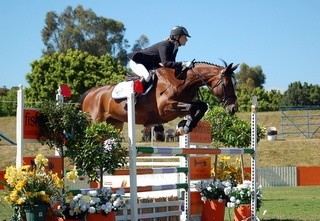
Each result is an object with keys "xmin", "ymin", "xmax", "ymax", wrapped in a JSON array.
[{"xmin": 181, "ymin": 62, "xmax": 193, "ymax": 68}]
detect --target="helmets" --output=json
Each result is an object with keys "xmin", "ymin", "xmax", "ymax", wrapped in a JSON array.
[{"xmin": 170, "ymin": 26, "xmax": 191, "ymax": 37}]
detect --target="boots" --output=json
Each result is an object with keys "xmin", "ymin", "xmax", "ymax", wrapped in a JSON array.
[{"xmin": 124, "ymin": 76, "xmax": 153, "ymax": 111}]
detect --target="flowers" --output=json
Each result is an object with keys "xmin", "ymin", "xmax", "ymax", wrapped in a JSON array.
[
  {"xmin": 0, "ymin": 153, "xmax": 127, "ymax": 221},
  {"xmin": 192, "ymin": 154, "xmax": 268, "ymax": 216}
]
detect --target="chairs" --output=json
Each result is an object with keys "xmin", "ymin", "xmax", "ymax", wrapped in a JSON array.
[{"xmin": 164, "ymin": 128, "xmax": 175, "ymax": 142}]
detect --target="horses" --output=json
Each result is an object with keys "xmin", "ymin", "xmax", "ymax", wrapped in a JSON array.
[{"xmin": 79, "ymin": 61, "xmax": 238, "ymax": 135}]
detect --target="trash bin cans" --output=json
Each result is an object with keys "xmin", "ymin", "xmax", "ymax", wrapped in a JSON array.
[{"xmin": 267, "ymin": 127, "xmax": 277, "ymax": 140}]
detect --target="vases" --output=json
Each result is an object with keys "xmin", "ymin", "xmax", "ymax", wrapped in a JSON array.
[
  {"xmin": 200, "ymin": 199, "xmax": 227, "ymax": 221},
  {"xmin": 84, "ymin": 209, "xmax": 116, "ymax": 221},
  {"xmin": 26, "ymin": 208, "xmax": 46, "ymax": 221},
  {"xmin": 234, "ymin": 203, "xmax": 251, "ymax": 221}
]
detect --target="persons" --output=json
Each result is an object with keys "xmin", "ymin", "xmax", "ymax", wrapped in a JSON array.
[
  {"xmin": 154, "ymin": 124, "xmax": 165, "ymax": 141},
  {"xmin": 145, "ymin": 127, "xmax": 151, "ymax": 142},
  {"xmin": 125, "ymin": 25, "xmax": 193, "ymax": 108}
]
